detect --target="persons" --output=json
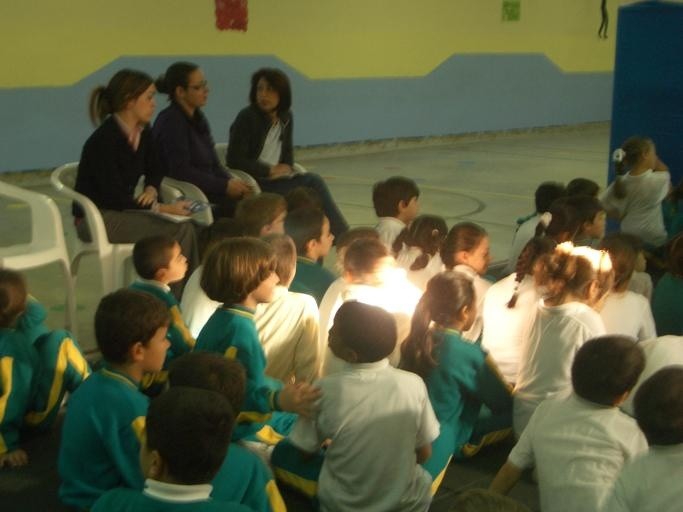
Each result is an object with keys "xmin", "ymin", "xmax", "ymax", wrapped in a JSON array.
[
  {"xmin": 155, "ymin": 62, "xmax": 252, "ymax": 217},
  {"xmin": 72, "ymin": 70, "xmax": 197, "ymax": 298},
  {"xmin": 601, "ymin": 137, "xmax": 669, "ymax": 244},
  {"xmin": 228, "ymin": 68, "xmax": 349, "ymax": 234},
  {"xmin": 0, "ymin": 179, "xmax": 683, "ymax": 512}
]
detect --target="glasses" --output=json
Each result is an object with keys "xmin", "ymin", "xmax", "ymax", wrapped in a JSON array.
[{"xmin": 188, "ymin": 79, "xmax": 208, "ymax": 89}]
